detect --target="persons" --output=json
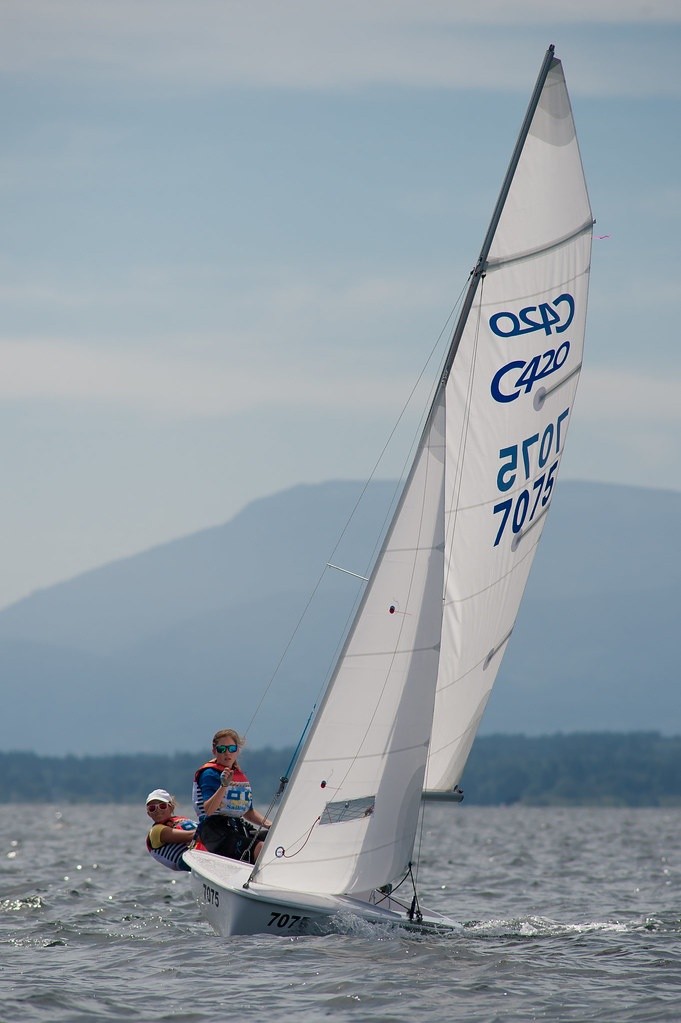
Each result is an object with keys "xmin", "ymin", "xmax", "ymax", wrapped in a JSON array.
[
  {"xmin": 145, "ymin": 789, "xmax": 199, "ymax": 871},
  {"xmin": 192, "ymin": 729, "xmax": 273, "ymax": 864}
]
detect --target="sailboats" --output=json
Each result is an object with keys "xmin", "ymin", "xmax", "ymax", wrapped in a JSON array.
[{"xmin": 182, "ymin": 41, "xmax": 598, "ymax": 939}]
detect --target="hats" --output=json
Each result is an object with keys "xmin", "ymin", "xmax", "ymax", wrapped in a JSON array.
[{"xmin": 145, "ymin": 789, "xmax": 172, "ymax": 805}]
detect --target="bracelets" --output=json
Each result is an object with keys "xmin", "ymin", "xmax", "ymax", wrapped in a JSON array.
[
  {"xmin": 268, "ymin": 825, "xmax": 271, "ymax": 829},
  {"xmin": 220, "ymin": 784, "xmax": 228, "ymax": 788}
]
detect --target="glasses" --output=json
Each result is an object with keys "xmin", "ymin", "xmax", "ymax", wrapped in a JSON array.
[
  {"xmin": 215, "ymin": 744, "xmax": 238, "ymax": 754},
  {"xmin": 147, "ymin": 801, "xmax": 172, "ymax": 814}
]
